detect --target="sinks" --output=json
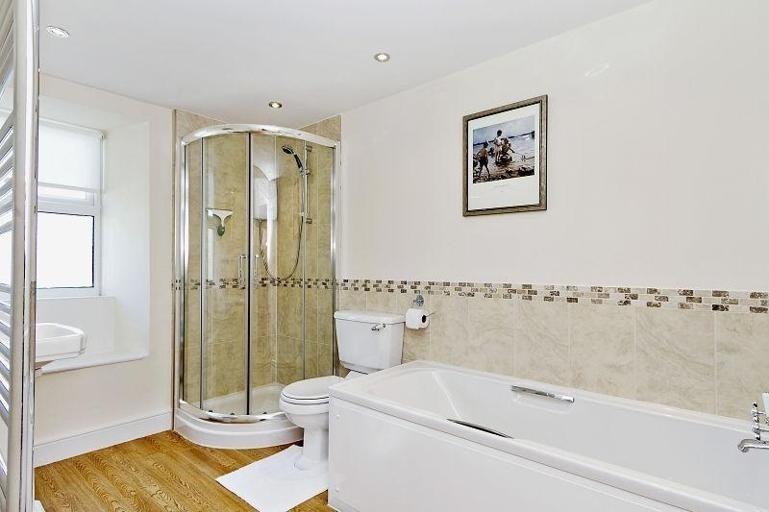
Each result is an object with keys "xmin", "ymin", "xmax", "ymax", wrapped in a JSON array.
[{"xmin": 35, "ymin": 322, "xmax": 87, "ymax": 365}]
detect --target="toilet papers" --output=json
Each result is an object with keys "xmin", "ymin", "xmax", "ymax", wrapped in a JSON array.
[{"xmin": 404, "ymin": 308, "xmax": 428, "ymax": 331}]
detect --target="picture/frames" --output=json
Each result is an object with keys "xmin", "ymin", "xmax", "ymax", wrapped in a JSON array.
[{"xmin": 461, "ymin": 94, "xmax": 548, "ymax": 218}]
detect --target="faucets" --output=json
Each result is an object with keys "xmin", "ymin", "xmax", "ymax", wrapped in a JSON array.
[{"xmin": 496, "ymin": 291, "xmax": 735, "ymax": 398}]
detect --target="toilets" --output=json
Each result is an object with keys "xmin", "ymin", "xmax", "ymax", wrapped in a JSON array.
[{"xmin": 279, "ymin": 309, "xmax": 408, "ymax": 473}]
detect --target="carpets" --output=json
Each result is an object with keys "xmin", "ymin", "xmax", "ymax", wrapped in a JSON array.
[{"xmin": 214, "ymin": 443, "xmax": 329, "ymax": 512}]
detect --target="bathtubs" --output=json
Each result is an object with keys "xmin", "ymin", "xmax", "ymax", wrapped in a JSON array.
[{"xmin": 324, "ymin": 359, "xmax": 765, "ymax": 512}]
